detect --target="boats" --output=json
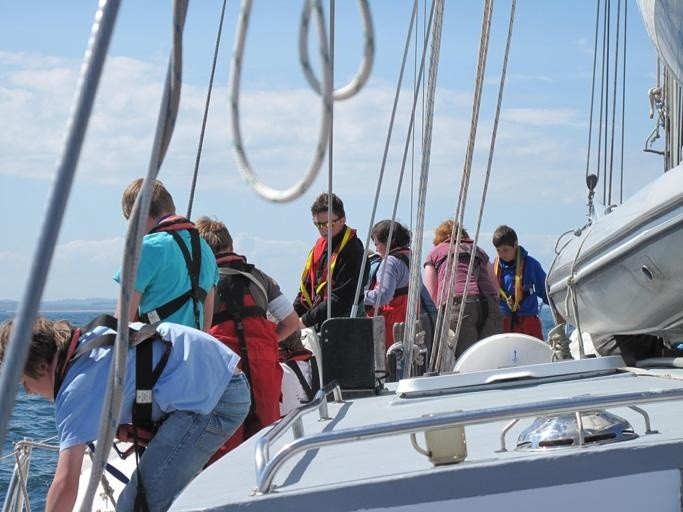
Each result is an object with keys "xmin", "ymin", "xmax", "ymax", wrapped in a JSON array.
[{"xmin": 528, "ymin": 150, "xmax": 682, "ymax": 339}]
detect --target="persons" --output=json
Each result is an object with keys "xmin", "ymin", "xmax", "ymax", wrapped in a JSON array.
[
  {"xmin": 291, "ymin": 192, "xmax": 370, "ymax": 398},
  {"xmin": 423, "ymin": 219, "xmax": 501, "ymax": 360},
  {"xmin": 107, "ymin": 177, "xmax": 219, "ymax": 335},
  {"xmin": 364, "ymin": 220, "xmax": 423, "ymax": 373},
  {"xmin": 491, "ymin": 225, "xmax": 565, "ymax": 341},
  {"xmin": 0, "ymin": 316, "xmax": 250, "ymax": 512},
  {"xmin": 194, "ymin": 215, "xmax": 300, "ymax": 470}
]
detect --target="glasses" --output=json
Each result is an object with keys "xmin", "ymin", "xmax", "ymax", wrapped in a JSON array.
[{"xmin": 314, "ymin": 218, "xmax": 343, "ymax": 229}]
